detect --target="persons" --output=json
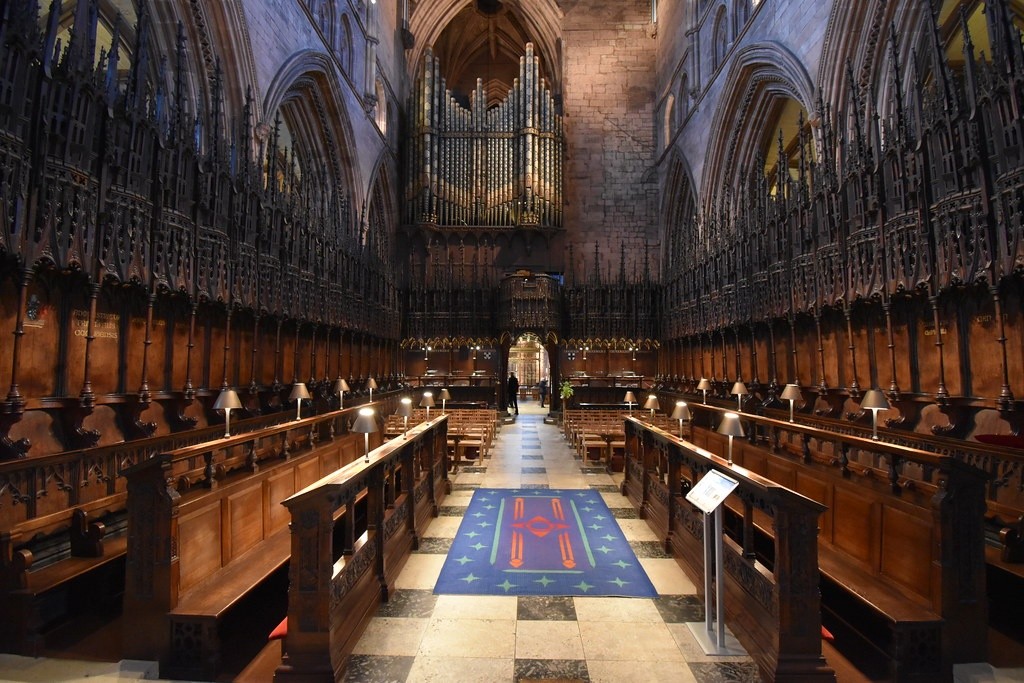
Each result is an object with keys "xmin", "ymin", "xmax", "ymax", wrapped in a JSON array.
[
  {"xmin": 538, "ymin": 378, "xmax": 548, "ymax": 408},
  {"xmin": 507, "ymin": 372, "xmax": 519, "ymax": 415}
]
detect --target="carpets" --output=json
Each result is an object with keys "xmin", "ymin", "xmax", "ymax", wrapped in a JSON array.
[{"xmin": 432, "ymin": 489, "xmax": 660, "ymax": 595}]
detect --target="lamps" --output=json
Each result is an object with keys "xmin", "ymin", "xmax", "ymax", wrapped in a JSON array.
[
  {"xmin": 860, "ymin": 390, "xmax": 890, "ymax": 442},
  {"xmin": 395, "ymin": 399, "xmax": 414, "ymax": 440},
  {"xmin": 351, "ymin": 412, "xmax": 379, "ymax": 463},
  {"xmin": 644, "ymin": 395, "xmax": 660, "ymax": 426},
  {"xmin": 779, "ymin": 384, "xmax": 803, "ymax": 423},
  {"xmin": 212, "ymin": 388, "xmax": 244, "ymax": 438},
  {"xmin": 717, "ymin": 413, "xmax": 745, "ymax": 465},
  {"xmin": 670, "ymin": 403, "xmax": 691, "ymax": 442},
  {"xmin": 697, "ymin": 379, "xmax": 712, "ymax": 405},
  {"xmin": 437, "ymin": 389, "xmax": 451, "ymax": 415},
  {"xmin": 365, "ymin": 378, "xmax": 377, "ymax": 403},
  {"xmin": 334, "ymin": 379, "xmax": 350, "ymax": 410},
  {"xmin": 730, "ymin": 381, "xmax": 749, "ymax": 412},
  {"xmin": 624, "ymin": 391, "xmax": 637, "ymax": 417},
  {"xmin": 287, "ymin": 383, "xmax": 310, "ymax": 422},
  {"xmin": 419, "ymin": 392, "xmax": 435, "ymax": 423}
]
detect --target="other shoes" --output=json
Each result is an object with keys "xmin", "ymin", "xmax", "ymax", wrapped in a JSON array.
[
  {"xmin": 514, "ymin": 412, "xmax": 519, "ymax": 415},
  {"xmin": 509, "ymin": 406, "xmax": 514, "ymax": 408},
  {"xmin": 541, "ymin": 406, "xmax": 545, "ymax": 408}
]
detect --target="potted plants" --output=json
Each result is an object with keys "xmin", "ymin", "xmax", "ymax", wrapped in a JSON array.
[{"xmin": 559, "ymin": 381, "xmax": 575, "ymax": 399}]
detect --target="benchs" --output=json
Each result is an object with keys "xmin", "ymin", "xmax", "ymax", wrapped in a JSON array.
[{"xmin": 383, "ymin": 407, "xmax": 691, "ymax": 468}]
